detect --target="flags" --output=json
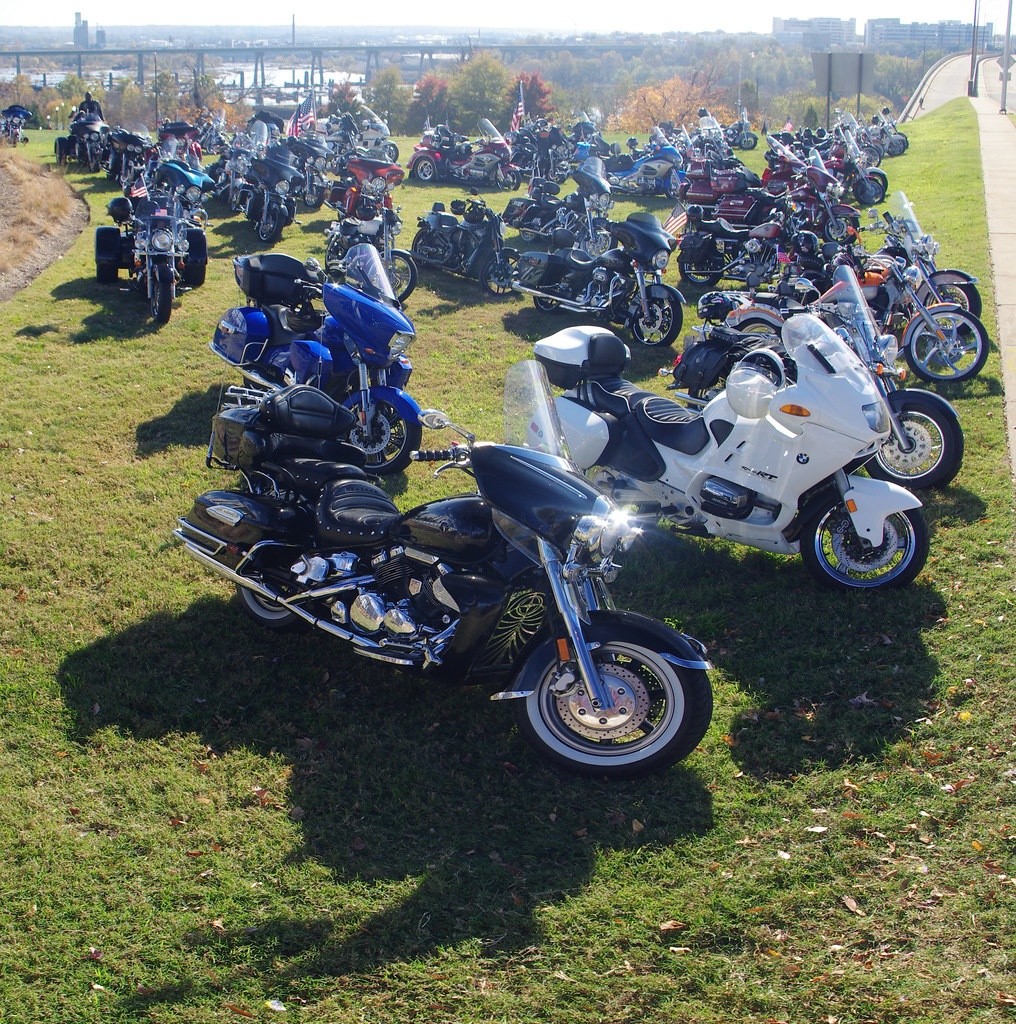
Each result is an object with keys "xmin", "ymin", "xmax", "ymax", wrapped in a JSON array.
[
  {"xmin": 663, "ymin": 201, "xmax": 688, "ymax": 236},
  {"xmin": 422, "ymin": 117, "xmax": 430, "ymax": 131},
  {"xmin": 780, "ymin": 117, "xmax": 794, "ymax": 136},
  {"xmin": 129, "ymin": 176, "xmax": 147, "ymax": 198},
  {"xmin": 287, "ymin": 91, "xmax": 318, "ymax": 140},
  {"xmin": 776, "ymin": 245, "xmax": 790, "ymax": 263},
  {"xmin": 512, "ymin": 83, "xmax": 524, "ymax": 131}
]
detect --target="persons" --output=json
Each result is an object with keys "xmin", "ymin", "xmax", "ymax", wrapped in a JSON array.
[{"xmin": 79, "ymin": 92, "xmax": 103, "ymax": 120}]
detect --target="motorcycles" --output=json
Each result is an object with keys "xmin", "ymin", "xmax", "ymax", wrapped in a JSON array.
[
  {"xmin": 171, "ymin": 358, "xmax": 717, "ymax": 786},
  {"xmin": 52, "ymin": 83, "xmax": 991, "ymax": 499},
  {"xmin": 519, "ymin": 312, "xmax": 931, "ymax": 598},
  {"xmin": 0, "ymin": 110, "xmax": 27, "ymax": 148},
  {"xmin": 208, "ymin": 244, "xmax": 424, "ymax": 479}
]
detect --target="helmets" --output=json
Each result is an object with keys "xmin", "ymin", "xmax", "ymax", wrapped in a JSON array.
[
  {"xmin": 627, "ymin": 137, "xmax": 638, "ymax": 148},
  {"xmin": 564, "ymin": 194, "xmax": 583, "ymax": 211},
  {"xmin": 882, "ymin": 107, "xmax": 889, "ymax": 114},
  {"xmin": 790, "ymin": 230, "xmax": 820, "ymax": 256},
  {"xmin": 109, "ymin": 197, "xmax": 134, "ymax": 222},
  {"xmin": 687, "ymin": 205, "xmax": 704, "ymax": 220},
  {"xmin": 816, "ymin": 127, "xmax": 826, "ymax": 137},
  {"xmin": 463, "ymin": 212, "xmax": 485, "ymax": 225},
  {"xmin": 803, "ymin": 127, "xmax": 812, "ymax": 137},
  {"xmin": 461, "ymin": 141, "xmax": 473, "ymax": 154},
  {"xmin": 726, "ymin": 348, "xmax": 787, "ymax": 421},
  {"xmin": 698, "ymin": 108, "xmax": 709, "ymax": 116},
  {"xmin": 284, "ymin": 308, "xmax": 325, "ymax": 332},
  {"xmin": 698, "ymin": 291, "xmax": 733, "ymax": 319},
  {"xmin": 356, "ymin": 206, "xmax": 375, "ymax": 221},
  {"xmin": 451, "ymin": 200, "xmax": 466, "ymax": 215},
  {"xmin": 871, "ymin": 115, "xmax": 879, "ymax": 124}
]
